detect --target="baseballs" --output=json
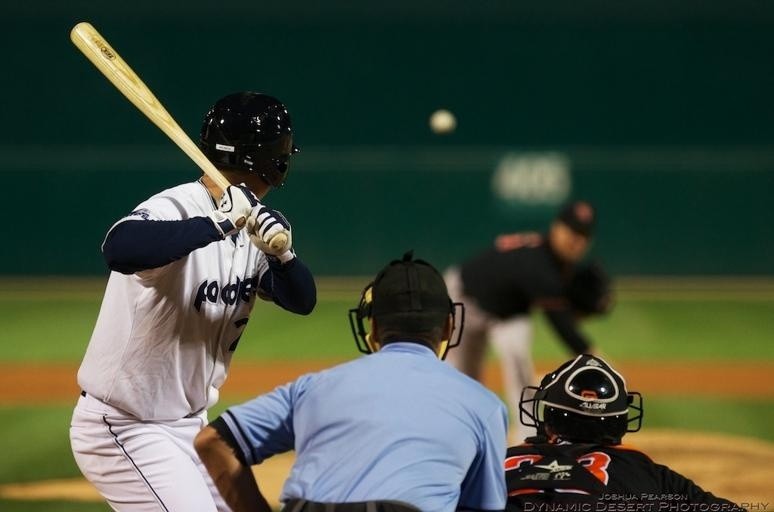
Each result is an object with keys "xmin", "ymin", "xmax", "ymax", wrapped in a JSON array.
[{"xmin": 430, "ymin": 110, "xmax": 458, "ymax": 135}]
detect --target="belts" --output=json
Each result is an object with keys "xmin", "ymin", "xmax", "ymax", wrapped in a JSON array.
[{"xmin": 280, "ymin": 498, "xmax": 417, "ymax": 512}]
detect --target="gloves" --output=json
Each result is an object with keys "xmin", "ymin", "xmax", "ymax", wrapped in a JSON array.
[
  {"xmin": 208, "ymin": 182, "xmax": 259, "ymax": 237},
  {"xmin": 246, "ymin": 201, "xmax": 296, "ymax": 266}
]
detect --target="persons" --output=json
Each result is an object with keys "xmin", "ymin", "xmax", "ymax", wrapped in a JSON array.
[
  {"xmin": 437, "ymin": 199, "xmax": 616, "ymax": 442},
  {"xmin": 66, "ymin": 90, "xmax": 317, "ymax": 511},
  {"xmin": 191, "ymin": 250, "xmax": 512, "ymax": 512},
  {"xmin": 500, "ymin": 354, "xmax": 747, "ymax": 512}
]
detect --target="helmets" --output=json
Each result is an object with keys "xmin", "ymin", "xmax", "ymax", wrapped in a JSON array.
[
  {"xmin": 518, "ymin": 352, "xmax": 642, "ymax": 444},
  {"xmin": 200, "ymin": 92, "xmax": 299, "ymax": 190},
  {"xmin": 349, "ymin": 249, "xmax": 465, "ymax": 363}
]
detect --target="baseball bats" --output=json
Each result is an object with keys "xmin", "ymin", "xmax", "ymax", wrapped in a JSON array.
[{"xmin": 69, "ymin": 23, "xmax": 285, "ymax": 252}]
